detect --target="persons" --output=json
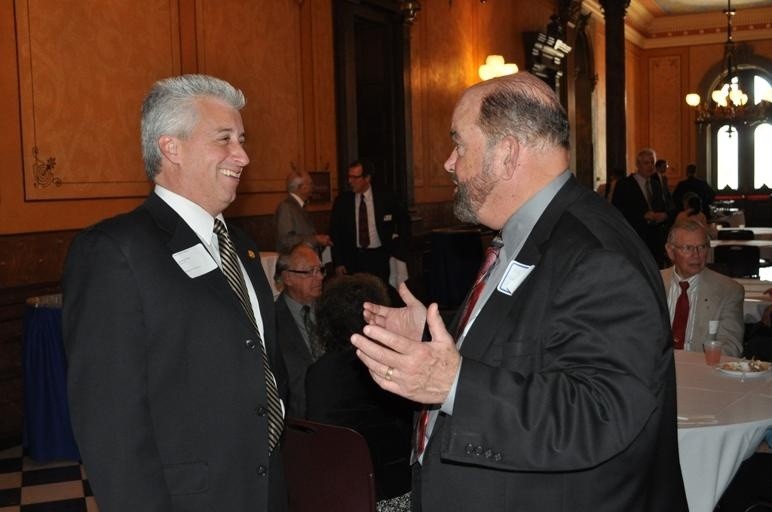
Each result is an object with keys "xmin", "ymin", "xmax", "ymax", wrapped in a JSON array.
[
  {"xmin": 742, "ymin": 287, "xmax": 771, "ymax": 362},
  {"xmin": 329, "ymin": 154, "xmax": 413, "ymax": 307},
  {"xmin": 348, "ymin": 71, "xmax": 689, "ymax": 512},
  {"xmin": 271, "ymin": 243, "xmax": 328, "ymax": 421},
  {"xmin": 57, "ymin": 74, "xmax": 291, "ymax": 511},
  {"xmin": 650, "ymin": 158, "xmax": 716, "ymax": 226},
  {"xmin": 611, "ymin": 147, "xmax": 669, "ymax": 248},
  {"xmin": 302, "ymin": 271, "xmax": 415, "ymax": 499},
  {"xmin": 273, "ymin": 169, "xmax": 334, "ymax": 257},
  {"xmin": 658, "ymin": 220, "xmax": 746, "ymax": 357}
]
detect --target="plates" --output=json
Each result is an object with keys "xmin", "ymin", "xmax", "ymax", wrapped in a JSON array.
[{"xmin": 719, "ymin": 360, "xmax": 770, "ymax": 378}]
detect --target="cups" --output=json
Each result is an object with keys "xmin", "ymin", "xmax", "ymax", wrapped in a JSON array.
[{"xmin": 704, "ymin": 341, "xmax": 721, "ymax": 365}]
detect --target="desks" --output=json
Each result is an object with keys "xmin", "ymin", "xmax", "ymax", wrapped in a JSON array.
[
  {"xmin": 674, "ymin": 207, "xmax": 772, "ymax": 512},
  {"xmin": 259, "ymin": 252, "xmax": 280, "ymax": 299},
  {"xmin": 22, "ymin": 293, "xmax": 83, "ymax": 462}
]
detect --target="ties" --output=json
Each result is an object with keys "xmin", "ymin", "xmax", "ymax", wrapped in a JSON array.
[
  {"xmin": 645, "ymin": 178, "xmax": 656, "ymax": 210},
  {"xmin": 360, "ymin": 194, "xmax": 371, "ymax": 251},
  {"xmin": 213, "ymin": 217, "xmax": 285, "ymax": 457},
  {"xmin": 300, "ymin": 304, "xmax": 327, "ymax": 362},
  {"xmin": 672, "ymin": 281, "xmax": 689, "ymax": 349},
  {"xmin": 410, "ymin": 230, "xmax": 504, "ymax": 466}
]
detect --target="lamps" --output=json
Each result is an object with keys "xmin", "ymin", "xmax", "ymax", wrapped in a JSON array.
[
  {"xmin": 685, "ymin": 0, "xmax": 772, "ymax": 133},
  {"xmin": 479, "ymin": 55, "xmax": 519, "ymax": 80}
]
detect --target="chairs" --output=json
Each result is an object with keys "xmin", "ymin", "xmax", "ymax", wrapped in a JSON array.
[{"xmin": 280, "ymin": 420, "xmax": 413, "ymax": 512}]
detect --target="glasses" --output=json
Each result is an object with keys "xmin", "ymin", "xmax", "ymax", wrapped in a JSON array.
[
  {"xmin": 285, "ymin": 266, "xmax": 328, "ymax": 280},
  {"xmin": 346, "ymin": 174, "xmax": 363, "ymax": 181},
  {"xmin": 671, "ymin": 240, "xmax": 710, "ymax": 255}
]
